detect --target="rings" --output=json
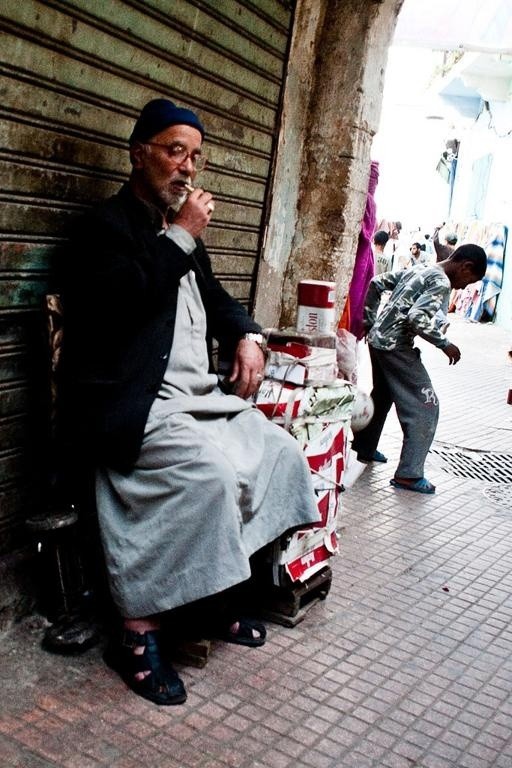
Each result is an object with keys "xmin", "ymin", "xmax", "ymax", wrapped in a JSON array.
[{"xmin": 256, "ymin": 373, "xmax": 262, "ymax": 379}]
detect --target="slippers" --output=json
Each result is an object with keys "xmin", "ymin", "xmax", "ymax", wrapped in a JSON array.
[
  {"xmin": 390, "ymin": 477, "xmax": 435, "ymax": 493},
  {"xmin": 365, "ymin": 451, "xmax": 388, "ymax": 462}
]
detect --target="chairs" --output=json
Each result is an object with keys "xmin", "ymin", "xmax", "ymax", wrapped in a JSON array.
[{"xmin": 43, "ymin": 290, "xmax": 215, "ymax": 670}]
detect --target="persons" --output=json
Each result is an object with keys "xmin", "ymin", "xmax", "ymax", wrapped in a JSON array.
[
  {"xmin": 428, "ymin": 221, "xmax": 457, "ymax": 335},
  {"xmin": 366, "ymin": 214, "xmax": 437, "ymax": 278},
  {"xmin": 49, "ymin": 88, "xmax": 315, "ymax": 708},
  {"xmin": 355, "ymin": 236, "xmax": 490, "ymax": 499}
]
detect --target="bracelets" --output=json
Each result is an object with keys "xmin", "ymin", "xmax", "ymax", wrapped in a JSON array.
[{"xmin": 242, "ymin": 331, "xmax": 268, "ymax": 354}]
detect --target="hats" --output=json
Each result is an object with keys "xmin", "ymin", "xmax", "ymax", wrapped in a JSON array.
[{"xmin": 128, "ymin": 98, "xmax": 204, "ymax": 147}]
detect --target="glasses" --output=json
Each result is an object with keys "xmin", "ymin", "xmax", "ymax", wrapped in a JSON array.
[{"xmin": 135, "ymin": 139, "xmax": 209, "ymax": 172}]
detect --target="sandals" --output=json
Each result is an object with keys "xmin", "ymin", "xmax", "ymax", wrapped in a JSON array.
[
  {"xmin": 103, "ymin": 628, "xmax": 187, "ymax": 704},
  {"xmin": 218, "ymin": 617, "xmax": 267, "ymax": 645}
]
